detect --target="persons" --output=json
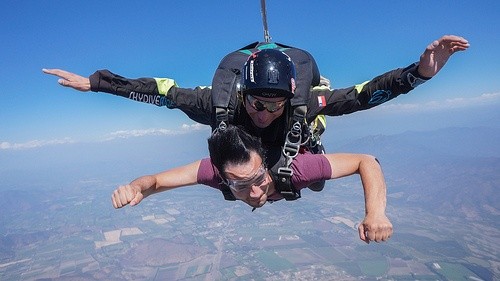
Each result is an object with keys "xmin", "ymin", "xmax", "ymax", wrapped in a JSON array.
[
  {"xmin": 42, "ymin": 35, "xmax": 470, "ymax": 155},
  {"xmin": 111, "ymin": 126, "xmax": 393, "ymax": 244}
]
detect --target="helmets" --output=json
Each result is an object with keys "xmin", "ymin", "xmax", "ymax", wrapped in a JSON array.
[{"xmin": 242, "ymin": 49, "xmax": 296, "ymax": 99}]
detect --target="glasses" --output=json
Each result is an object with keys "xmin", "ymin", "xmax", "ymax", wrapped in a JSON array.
[
  {"xmin": 221, "ymin": 164, "xmax": 266, "ymax": 192},
  {"xmin": 247, "ymin": 95, "xmax": 287, "ymax": 112}
]
